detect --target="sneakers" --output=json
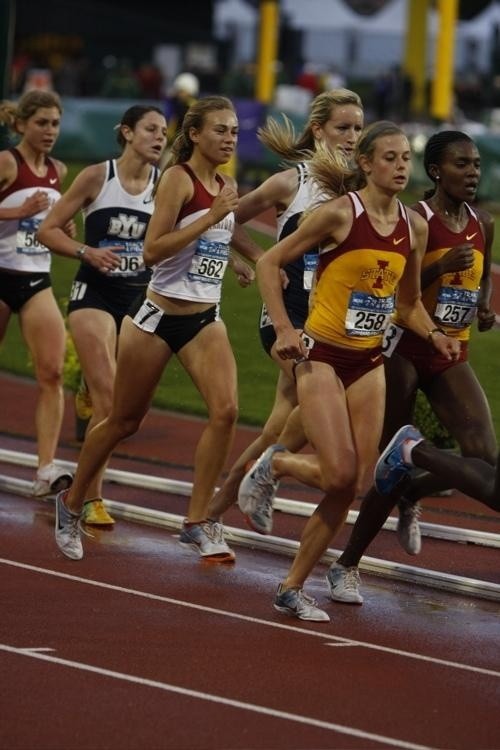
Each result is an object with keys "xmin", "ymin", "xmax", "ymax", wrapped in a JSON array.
[
  {"xmin": 397, "ymin": 497, "xmax": 423, "ymax": 556},
  {"xmin": 32, "ymin": 463, "xmax": 116, "ymax": 561},
  {"xmin": 326, "ymin": 555, "xmax": 363, "ymax": 604},
  {"xmin": 374, "ymin": 425, "xmax": 425, "ymax": 497},
  {"xmin": 238, "ymin": 444, "xmax": 286, "ymax": 535},
  {"xmin": 273, "ymin": 582, "xmax": 330, "ymax": 622},
  {"xmin": 75, "ymin": 371, "xmax": 93, "ymax": 421},
  {"xmin": 175, "ymin": 517, "xmax": 236, "ymax": 562}
]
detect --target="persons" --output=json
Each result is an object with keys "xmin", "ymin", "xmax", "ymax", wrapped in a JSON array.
[
  {"xmin": 37, "ymin": 106, "xmax": 168, "ymax": 528},
  {"xmin": 0, "ymin": 90, "xmax": 78, "ymax": 494},
  {"xmin": 207, "ymin": 90, "xmax": 366, "ymax": 567},
  {"xmin": 453, "ymin": 61, "xmax": 495, "ymax": 122},
  {"xmin": 9, "ymin": 30, "xmax": 183, "ymax": 97},
  {"xmin": 326, "ymin": 133, "xmax": 500, "ymax": 607},
  {"xmin": 56, "ymin": 94, "xmax": 256, "ymax": 560},
  {"xmin": 371, "ymin": 423, "xmax": 500, "ymax": 514},
  {"xmin": 255, "ymin": 118, "xmax": 462, "ymax": 623},
  {"xmin": 376, "ymin": 64, "xmax": 414, "ymax": 123}
]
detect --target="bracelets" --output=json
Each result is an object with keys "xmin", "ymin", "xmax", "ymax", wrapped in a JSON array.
[
  {"xmin": 428, "ymin": 328, "xmax": 445, "ymax": 345},
  {"xmin": 76, "ymin": 244, "xmax": 89, "ymax": 261}
]
